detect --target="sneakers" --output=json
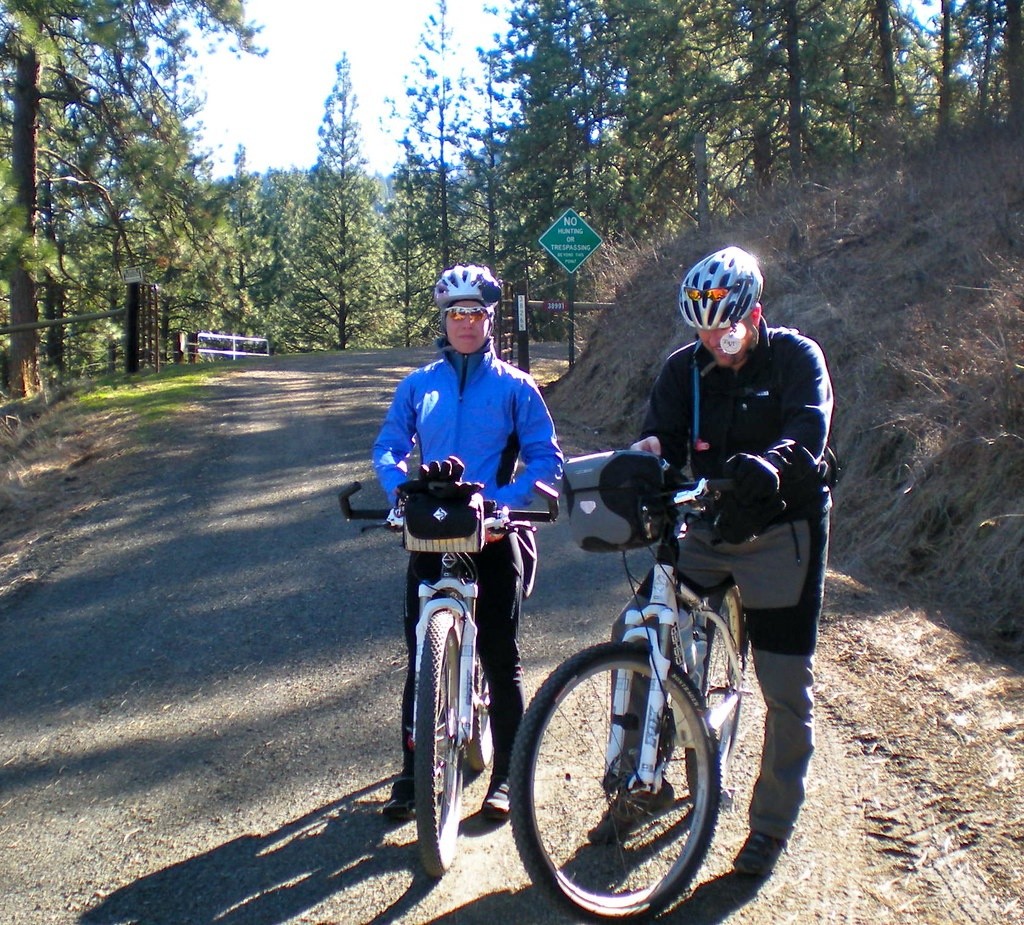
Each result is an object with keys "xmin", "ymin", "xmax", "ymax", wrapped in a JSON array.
[
  {"xmin": 482, "ymin": 774, "xmax": 511, "ymax": 820},
  {"xmin": 383, "ymin": 772, "xmax": 417, "ymax": 818},
  {"xmin": 734, "ymin": 832, "xmax": 786, "ymax": 879},
  {"xmin": 587, "ymin": 777, "xmax": 675, "ymax": 846}
]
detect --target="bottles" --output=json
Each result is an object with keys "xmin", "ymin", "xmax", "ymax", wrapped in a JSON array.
[{"xmin": 676, "ymin": 605, "xmax": 708, "ymax": 690}]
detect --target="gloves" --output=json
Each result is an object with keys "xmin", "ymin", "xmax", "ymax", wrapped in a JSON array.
[
  {"xmin": 400, "ymin": 455, "xmax": 484, "ymax": 502},
  {"xmin": 726, "ymin": 452, "xmax": 780, "ymax": 507}
]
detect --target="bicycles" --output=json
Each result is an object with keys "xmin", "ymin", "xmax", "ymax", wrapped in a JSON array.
[
  {"xmin": 339, "ymin": 482, "xmax": 561, "ymax": 879},
  {"xmin": 507, "ymin": 473, "xmax": 747, "ymax": 925}
]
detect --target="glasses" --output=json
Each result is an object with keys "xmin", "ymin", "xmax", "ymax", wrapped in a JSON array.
[
  {"xmin": 442, "ymin": 306, "xmax": 488, "ymax": 322},
  {"xmin": 683, "ymin": 282, "xmax": 741, "ymax": 302}
]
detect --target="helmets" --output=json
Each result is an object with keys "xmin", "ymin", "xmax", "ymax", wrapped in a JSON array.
[
  {"xmin": 679, "ymin": 245, "xmax": 764, "ymax": 330},
  {"xmin": 433, "ymin": 265, "xmax": 502, "ymax": 309}
]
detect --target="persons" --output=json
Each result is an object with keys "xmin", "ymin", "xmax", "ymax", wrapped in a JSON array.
[
  {"xmin": 587, "ymin": 246, "xmax": 834, "ymax": 876},
  {"xmin": 371, "ymin": 265, "xmax": 565, "ymax": 819}
]
